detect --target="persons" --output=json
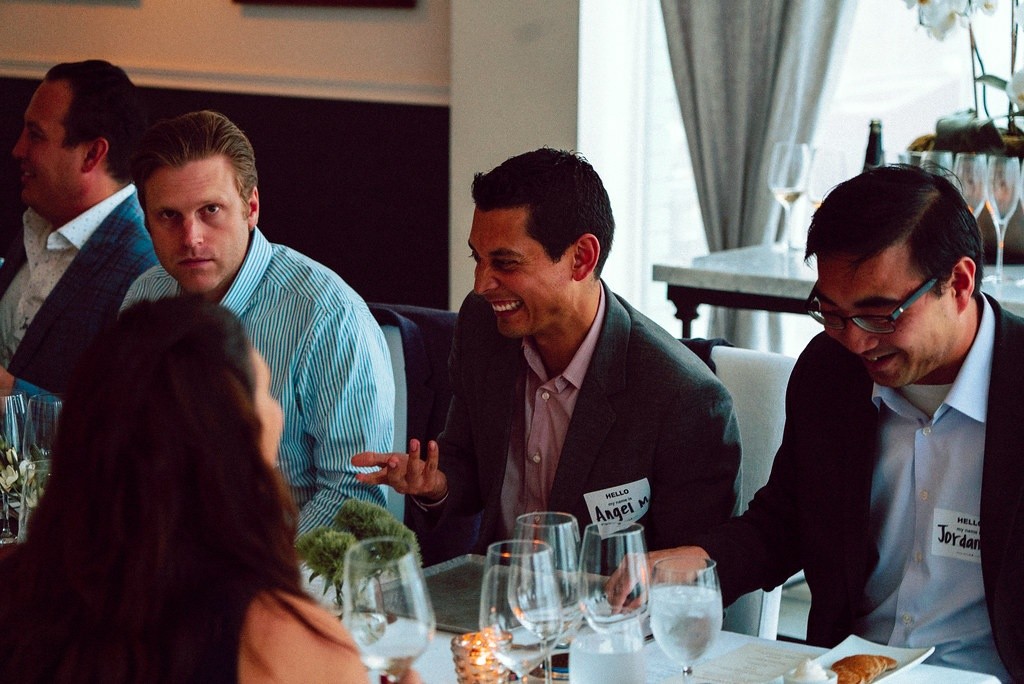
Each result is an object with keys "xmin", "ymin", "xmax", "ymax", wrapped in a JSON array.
[
  {"xmin": 0, "ymin": 296, "xmax": 373, "ymax": 684},
  {"xmin": 0, "ymin": 60, "xmax": 395, "ymax": 554},
  {"xmin": 350, "ymin": 147, "xmax": 744, "ymax": 620},
  {"xmin": 602, "ymin": 162, "xmax": 1024, "ymax": 684}
]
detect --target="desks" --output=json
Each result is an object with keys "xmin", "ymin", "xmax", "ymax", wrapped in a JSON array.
[
  {"xmin": 299, "ymin": 557, "xmax": 1002, "ymax": 684},
  {"xmin": 652, "ymin": 241, "xmax": 1024, "ymax": 339}
]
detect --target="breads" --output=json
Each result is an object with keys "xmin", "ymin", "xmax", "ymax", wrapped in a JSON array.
[{"xmin": 830, "ymin": 654, "xmax": 898, "ymax": 684}]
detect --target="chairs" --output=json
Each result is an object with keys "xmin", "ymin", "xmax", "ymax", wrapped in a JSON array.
[
  {"xmin": 711, "ymin": 345, "xmax": 798, "ymax": 639},
  {"xmin": 366, "ymin": 299, "xmax": 457, "ymax": 529}
]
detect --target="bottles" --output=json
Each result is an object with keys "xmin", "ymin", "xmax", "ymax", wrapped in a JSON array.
[{"xmin": 860, "ymin": 119, "xmax": 886, "ymax": 172}]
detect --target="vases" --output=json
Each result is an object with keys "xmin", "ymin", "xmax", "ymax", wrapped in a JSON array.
[{"xmin": 907, "ymin": 134, "xmax": 1024, "ymax": 265}]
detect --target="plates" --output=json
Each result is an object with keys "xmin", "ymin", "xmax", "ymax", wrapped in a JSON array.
[{"xmin": 770, "ymin": 634, "xmax": 935, "ymax": 684}]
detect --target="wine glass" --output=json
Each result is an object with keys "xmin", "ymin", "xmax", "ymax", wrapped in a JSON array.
[
  {"xmin": 507, "ymin": 511, "xmax": 589, "ymax": 684},
  {"xmin": 648, "ymin": 554, "xmax": 723, "ymax": 684},
  {"xmin": 478, "ymin": 538, "xmax": 563, "ymax": 684},
  {"xmin": 340, "ymin": 536, "xmax": 436, "ymax": 684},
  {"xmin": 896, "ymin": 150, "xmax": 1024, "ymax": 288},
  {"xmin": 766, "ymin": 141, "xmax": 811, "ymax": 257},
  {"xmin": 0, "ymin": 387, "xmax": 28, "ymax": 544}
]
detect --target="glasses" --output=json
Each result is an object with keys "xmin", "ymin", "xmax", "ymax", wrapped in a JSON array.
[{"xmin": 805, "ymin": 276, "xmax": 938, "ymax": 335}]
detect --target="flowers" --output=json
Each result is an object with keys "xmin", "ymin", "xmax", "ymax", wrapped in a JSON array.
[
  {"xmin": 903, "ymin": 0, "xmax": 1023, "ymax": 152},
  {"xmin": 0, "ymin": 436, "xmax": 46, "ymax": 521}
]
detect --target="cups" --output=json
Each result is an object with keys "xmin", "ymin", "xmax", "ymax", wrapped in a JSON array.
[
  {"xmin": 16, "ymin": 459, "xmax": 51, "ymax": 543},
  {"xmin": 22, "ymin": 392, "xmax": 62, "ymax": 471},
  {"xmin": 805, "ymin": 145, "xmax": 848, "ymax": 208},
  {"xmin": 575, "ymin": 520, "xmax": 650, "ymax": 635},
  {"xmin": 568, "ymin": 605, "xmax": 649, "ymax": 684}
]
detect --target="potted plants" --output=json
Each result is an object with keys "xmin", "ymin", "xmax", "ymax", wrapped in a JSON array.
[{"xmin": 295, "ymin": 498, "xmax": 424, "ymax": 645}]
